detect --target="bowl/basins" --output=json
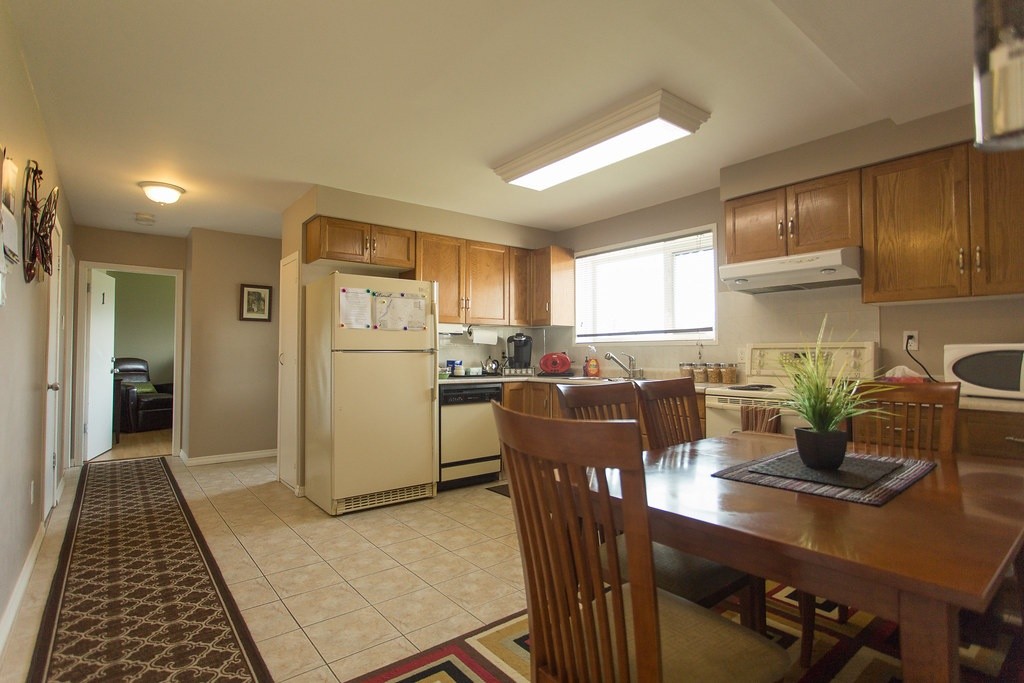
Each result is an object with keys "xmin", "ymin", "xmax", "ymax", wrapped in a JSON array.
[{"xmin": 439, "ymin": 373, "xmax": 449, "ymax": 379}]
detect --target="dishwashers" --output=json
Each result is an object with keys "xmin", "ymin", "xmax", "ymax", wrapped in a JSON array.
[{"xmin": 438, "ymin": 383, "xmax": 501, "ymax": 493}]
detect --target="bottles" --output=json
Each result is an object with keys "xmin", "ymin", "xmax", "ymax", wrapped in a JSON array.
[
  {"xmin": 706, "ymin": 363, "xmax": 721, "ymax": 383},
  {"xmin": 679, "ymin": 362, "xmax": 693, "ymax": 383},
  {"xmin": 691, "ymin": 363, "xmax": 707, "ymax": 383},
  {"xmin": 720, "ymin": 363, "xmax": 738, "ymax": 384}
]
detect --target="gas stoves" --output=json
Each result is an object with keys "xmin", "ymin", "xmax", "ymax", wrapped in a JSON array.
[{"xmin": 705, "ymin": 341, "xmax": 878, "ymax": 402}]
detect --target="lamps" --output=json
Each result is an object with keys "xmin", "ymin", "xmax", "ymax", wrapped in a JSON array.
[
  {"xmin": 490, "ymin": 90, "xmax": 710, "ymax": 191},
  {"xmin": 136, "ymin": 181, "xmax": 186, "ymax": 204}
]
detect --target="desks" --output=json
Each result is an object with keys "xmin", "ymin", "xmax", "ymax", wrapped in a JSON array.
[{"xmin": 544, "ymin": 430, "xmax": 1024, "ymax": 683}]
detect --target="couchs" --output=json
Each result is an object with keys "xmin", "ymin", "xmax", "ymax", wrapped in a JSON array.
[{"xmin": 114, "ymin": 358, "xmax": 173, "ymax": 432}]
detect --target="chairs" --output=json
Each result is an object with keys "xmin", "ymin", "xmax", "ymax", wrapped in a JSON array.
[
  {"xmin": 798, "ymin": 378, "xmax": 960, "ymax": 671},
  {"xmin": 491, "ymin": 399, "xmax": 794, "ymax": 682},
  {"xmin": 634, "ymin": 377, "xmax": 703, "ymax": 449},
  {"xmin": 555, "ymin": 382, "xmax": 769, "ymax": 636}
]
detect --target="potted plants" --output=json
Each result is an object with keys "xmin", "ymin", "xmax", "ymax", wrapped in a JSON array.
[{"xmin": 745, "ymin": 309, "xmax": 905, "ymax": 470}]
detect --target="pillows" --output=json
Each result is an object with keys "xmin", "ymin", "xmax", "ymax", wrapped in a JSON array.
[{"xmin": 124, "ymin": 382, "xmax": 157, "ymax": 392}]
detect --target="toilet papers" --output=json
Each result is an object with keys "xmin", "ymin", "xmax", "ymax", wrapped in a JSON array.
[{"xmin": 467, "ymin": 328, "xmax": 497, "ymax": 345}]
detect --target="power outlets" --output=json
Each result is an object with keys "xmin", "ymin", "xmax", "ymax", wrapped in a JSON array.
[
  {"xmin": 30, "ymin": 480, "xmax": 36, "ymax": 504},
  {"xmin": 737, "ymin": 348, "xmax": 746, "ymax": 364},
  {"xmin": 903, "ymin": 330, "xmax": 919, "ymax": 351}
]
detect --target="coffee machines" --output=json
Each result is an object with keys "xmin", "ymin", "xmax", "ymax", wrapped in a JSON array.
[{"xmin": 507, "ymin": 333, "xmax": 532, "ymax": 368}]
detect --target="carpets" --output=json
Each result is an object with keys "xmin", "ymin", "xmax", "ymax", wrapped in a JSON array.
[{"xmin": 345, "ymin": 561, "xmax": 1024, "ymax": 683}]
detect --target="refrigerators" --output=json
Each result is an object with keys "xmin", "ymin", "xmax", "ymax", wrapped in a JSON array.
[{"xmin": 304, "ymin": 271, "xmax": 438, "ymax": 516}]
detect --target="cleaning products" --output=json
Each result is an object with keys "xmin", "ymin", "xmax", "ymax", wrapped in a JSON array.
[{"xmin": 583, "ymin": 345, "xmax": 599, "ymax": 377}]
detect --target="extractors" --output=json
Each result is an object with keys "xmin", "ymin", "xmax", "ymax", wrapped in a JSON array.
[{"xmin": 718, "ymin": 247, "xmax": 863, "ymax": 295}]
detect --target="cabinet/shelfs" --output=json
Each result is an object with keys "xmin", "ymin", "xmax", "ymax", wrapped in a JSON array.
[
  {"xmin": 417, "ymin": 230, "xmax": 510, "ymax": 326},
  {"xmin": 725, "ymin": 170, "xmax": 859, "ymax": 264},
  {"xmin": 530, "ymin": 383, "xmax": 552, "ymax": 418},
  {"xmin": 532, "ymin": 247, "xmax": 552, "ymax": 325},
  {"xmin": 861, "ymin": 140, "xmax": 1024, "ymax": 305},
  {"xmin": 509, "ymin": 247, "xmax": 532, "ymax": 326},
  {"xmin": 320, "ymin": 216, "xmax": 415, "ymax": 270}
]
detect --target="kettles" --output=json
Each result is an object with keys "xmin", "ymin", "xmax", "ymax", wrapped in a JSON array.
[{"xmin": 480, "ymin": 356, "xmax": 499, "ymax": 376}]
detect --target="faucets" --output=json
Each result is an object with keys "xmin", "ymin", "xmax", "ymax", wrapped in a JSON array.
[{"xmin": 604, "ymin": 352, "xmax": 636, "ymax": 378}]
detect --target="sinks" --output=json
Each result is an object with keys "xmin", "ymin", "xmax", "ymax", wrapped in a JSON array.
[
  {"xmin": 568, "ymin": 377, "xmax": 607, "ymax": 380},
  {"xmin": 603, "ymin": 378, "xmax": 637, "ymax": 383}
]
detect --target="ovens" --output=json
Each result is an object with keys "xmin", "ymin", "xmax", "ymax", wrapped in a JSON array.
[{"xmin": 705, "ymin": 395, "xmax": 826, "ymax": 439}]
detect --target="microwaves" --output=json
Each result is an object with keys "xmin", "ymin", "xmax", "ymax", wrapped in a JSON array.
[{"xmin": 943, "ymin": 343, "xmax": 1024, "ymax": 400}]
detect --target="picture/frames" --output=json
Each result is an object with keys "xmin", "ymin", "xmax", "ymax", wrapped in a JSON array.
[{"xmin": 240, "ymin": 283, "xmax": 272, "ymax": 322}]
